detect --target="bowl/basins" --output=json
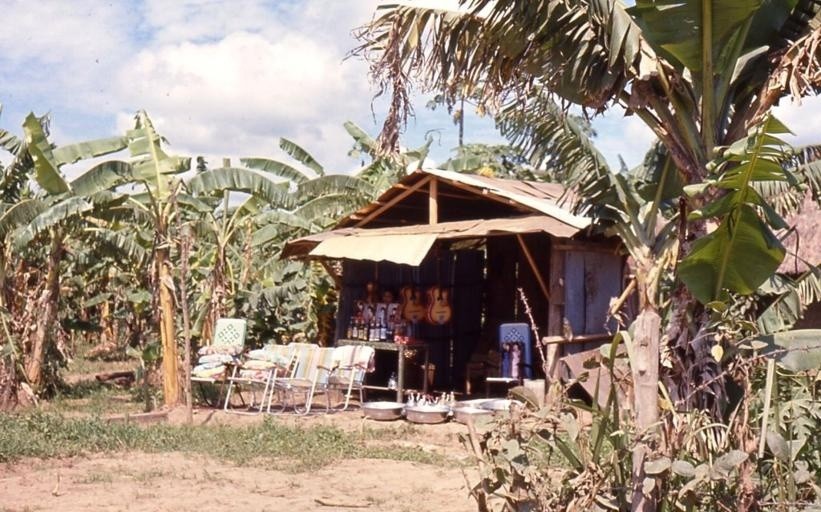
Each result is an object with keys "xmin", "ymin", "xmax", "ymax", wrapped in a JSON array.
[
  {"xmin": 478, "ymin": 396, "xmax": 522, "ymax": 417},
  {"xmin": 401, "ymin": 400, "xmax": 450, "ymax": 422},
  {"xmin": 362, "ymin": 397, "xmax": 406, "ymax": 422}
]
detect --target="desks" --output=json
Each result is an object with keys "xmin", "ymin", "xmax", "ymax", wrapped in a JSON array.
[{"xmin": 337, "ymin": 338, "xmax": 431, "ymax": 403}]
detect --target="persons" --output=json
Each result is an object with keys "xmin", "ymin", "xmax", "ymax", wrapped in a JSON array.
[{"xmin": 375, "ymin": 290, "xmax": 401, "ymax": 320}]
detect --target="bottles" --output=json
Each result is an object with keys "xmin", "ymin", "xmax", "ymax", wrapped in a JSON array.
[{"xmin": 346, "ymin": 316, "xmax": 387, "ymax": 342}]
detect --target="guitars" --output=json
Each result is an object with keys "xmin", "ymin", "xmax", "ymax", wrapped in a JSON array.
[
  {"xmin": 398, "ymin": 258, "xmax": 451, "ymax": 326},
  {"xmin": 366, "ymin": 263, "xmax": 382, "ymax": 303}
]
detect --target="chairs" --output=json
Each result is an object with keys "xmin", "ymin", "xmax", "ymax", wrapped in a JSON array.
[
  {"xmin": 191, "ymin": 317, "xmax": 373, "ymax": 417},
  {"xmin": 485, "ymin": 341, "xmax": 525, "ymax": 398}
]
